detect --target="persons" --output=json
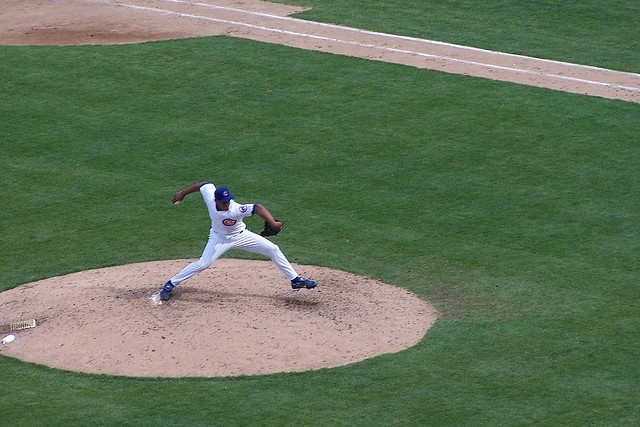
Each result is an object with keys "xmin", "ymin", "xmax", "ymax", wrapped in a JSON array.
[{"xmin": 160, "ymin": 181, "xmax": 317, "ymax": 300}]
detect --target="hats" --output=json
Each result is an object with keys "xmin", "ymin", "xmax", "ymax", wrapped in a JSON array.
[{"xmin": 214, "ymin": 188, "xmax": 233, "ymax": 202}]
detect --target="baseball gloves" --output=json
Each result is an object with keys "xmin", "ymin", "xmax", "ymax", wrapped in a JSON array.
[{"xmin": 260, "ymin": 219, "xmax": 283, "ymax": 237}]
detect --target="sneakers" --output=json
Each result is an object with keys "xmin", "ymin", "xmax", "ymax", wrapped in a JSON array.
[
  {"xmin": 160, "ymin": 281, "xmax": 175, "ymax": 301},
  {"xmin": 291, "ymin": 276, "xmax": 317, "ymax": 290}
]
